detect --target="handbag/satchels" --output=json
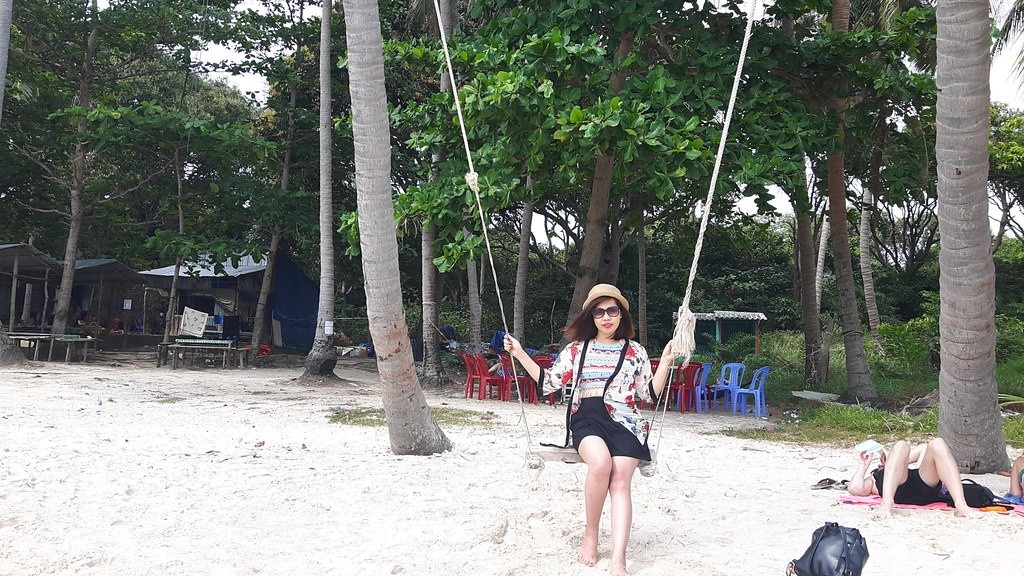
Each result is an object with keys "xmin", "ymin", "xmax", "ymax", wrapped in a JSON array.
[{"xmin": 946, "ymin": 480, "xmax": 993, "ymax": 508}]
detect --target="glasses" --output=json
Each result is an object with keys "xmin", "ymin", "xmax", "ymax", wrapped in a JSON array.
[{"xmin": 589, "ymin": 306, "xmax": 622, "ymax": 318}]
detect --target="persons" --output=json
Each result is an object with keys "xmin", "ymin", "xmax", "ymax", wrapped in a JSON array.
[
  {"xmin": 1008, "ymin": 455, "xmax": 1024, "ymax": 497},
  {"xmin": 503, "ymin": 284, "xmax": 678, "ymax": 576},
  {"xmin": 848, "ymin": 437, "xmax": 984, "ymax": 521}
]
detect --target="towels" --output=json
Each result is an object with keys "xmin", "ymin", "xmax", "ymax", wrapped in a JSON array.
[
  {"xmin": 123, "ymin": 299, "xmax": 131, "ymax": 309},
  {"xmin": 178, "ymin": 306, "xmax": 208, "ymax": 337},
  {"xmin": 222, "ymin": 316, "xmax": 239, "ymax": 340},
  {"xmin": 490, "ymin": 331, "xmax": 514, "ymax": 354}
]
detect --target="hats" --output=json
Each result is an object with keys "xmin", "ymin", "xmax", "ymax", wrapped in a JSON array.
[{"xmin": 582, "ymin": 284, "xmax": 629, "ymax": 310}]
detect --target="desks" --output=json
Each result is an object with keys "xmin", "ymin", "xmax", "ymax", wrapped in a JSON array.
[
  {"xmin": 173, "ymin": 339, "xmax": 233, "ymax": 370},
  {"xmin": 6, "ymin": 332, "xmax": 95, "ymax": 364}
]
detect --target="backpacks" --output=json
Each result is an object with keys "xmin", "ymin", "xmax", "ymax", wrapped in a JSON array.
[{"xmin": 787, "ymin": 523, "xmax": 868, "ymax": 576}]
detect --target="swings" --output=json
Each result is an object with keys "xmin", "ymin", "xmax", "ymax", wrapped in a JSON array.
[{"xmin": 433, "ymin": 0, "xmax": 759, "ymax": 478}]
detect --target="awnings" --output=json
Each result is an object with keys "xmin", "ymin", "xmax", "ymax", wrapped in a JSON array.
[{"xmin": 137, "ymin": 264, "xmax": 267, "ymax": 288}]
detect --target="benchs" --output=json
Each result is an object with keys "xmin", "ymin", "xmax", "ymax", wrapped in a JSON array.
[
  {"xmin": 174, "ymin": 346, "xmax": 251, "ymax": 370},
  {"xmin": 173, "ymin": 314, "xmax": 223, "ymax": 334}
]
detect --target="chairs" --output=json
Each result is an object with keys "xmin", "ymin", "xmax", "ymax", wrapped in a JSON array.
[
  {"xmin": 637, "ymin": 359, "xmax": 771, "ymax": 417},
  {"xmin": 460, "ymin": 352, "xmax": 573, "ymax": 406}
]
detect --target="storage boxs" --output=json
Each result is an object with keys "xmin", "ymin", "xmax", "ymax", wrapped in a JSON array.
[{"xmin": 355, "ymin": 349, "xmax": 368, "ymax": 357}]
